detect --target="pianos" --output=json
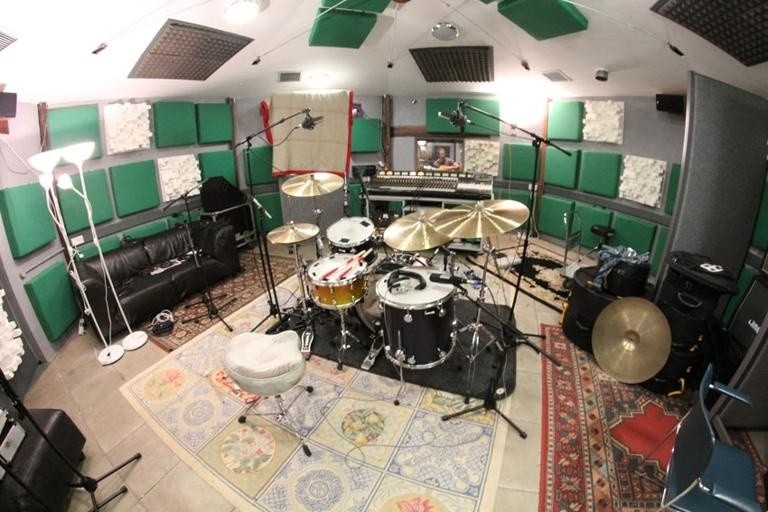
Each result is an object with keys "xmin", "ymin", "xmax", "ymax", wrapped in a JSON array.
[{"xmin": 361, "ymin": 176, "xmax": 493, "ymax": 204}]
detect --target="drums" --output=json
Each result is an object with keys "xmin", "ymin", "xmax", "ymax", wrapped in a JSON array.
[
  {"xmin": 355, "ymin": 253, "xmax": 437, "ymax": 337},
  {"xmin": 375, "ymin": 266, "xmax": 457, "ymax": 370},
  {"xmin": 326, "ymin": 216, "xmax": 378, "ymax": 266},
  {"xmin": 305, "ymin": 252, "xmax": 368, "ymax": 310}
]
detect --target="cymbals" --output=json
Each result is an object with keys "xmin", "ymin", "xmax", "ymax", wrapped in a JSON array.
[
  {"xmin": 434, "ymin": 199, "xmax": 531, "ymax": 238},
  {"xmin": 592, "ymin": 298, "xmax": 672, "ymax": 383},
  {"xmin": 266, "ymin": 222, "xmax": 320, "ymax": 244},
  {"xmin": 281, "ymin": 172, "xmax": 345, "ymax": 196},
  {"xmin": 384, "ymin": 208, "xmax": 450, "ymax": 252}
]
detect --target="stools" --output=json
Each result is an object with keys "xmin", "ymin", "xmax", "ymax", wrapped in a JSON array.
[{"xmin": 0, "ymin": 409, "xmax": 86, "ymax": 512}]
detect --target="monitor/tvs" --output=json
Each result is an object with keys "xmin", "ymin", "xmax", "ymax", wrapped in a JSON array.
[{"xmin": 415, "ymin": 137, "xmax": 464, "ymax": 173}]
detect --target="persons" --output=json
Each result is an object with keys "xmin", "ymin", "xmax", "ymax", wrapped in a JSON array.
[{"xmin": 434, "ymin": 148, "xmax": 459, "ymax": 173}]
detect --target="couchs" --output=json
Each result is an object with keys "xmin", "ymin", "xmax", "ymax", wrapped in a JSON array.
[{"xmin": 71, "ymin": 220, "xmax": 243, "ymax": 343}]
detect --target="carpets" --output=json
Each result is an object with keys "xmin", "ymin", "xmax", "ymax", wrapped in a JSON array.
[
  {"xmin": 467, "ymin": 243, "xmax": 585, "ymax": 314},
  {"xmin": 118, "ymin": 252, "xmax": 507, "ymax": 512},
  {"xmin": 541, "ymin": 324, "xmax": 768, "ymax": 512},
  {"xmin": 132, "ymin": 251, "xmax": 298, "ymax": 355}
]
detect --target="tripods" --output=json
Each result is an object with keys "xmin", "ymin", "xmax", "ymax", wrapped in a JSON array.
[
  {"xmin": 0, "ymin": 372, "xmax": 141, "ymax": 512},
  {"xmin": 181, "ymin": 196, "xmax": 238, "ymax": 332},
  {"xmin": 249, "ymin": 216, "xmax": 305, "ymax": 335},
  {"xmin": 0, "ymin": 486, "xmax": 133, "ymax": 512},
  {"xmin": 474, "ymin": 140, "xmax": 546, "ymax": 355},
  {"xmin": 318, "ymin": 302, "xmax": 382, "ymax": 370},
  {"xmin": 238, "ymin": 142, "xmax": 305, "ymax": 331},
  {"xmin": 290, "ymin": 245, "xmax": 325, "ymax": 336},
  {"xmin": 441, "ymin": 328, "xmax": 527, "ymax": 438}
]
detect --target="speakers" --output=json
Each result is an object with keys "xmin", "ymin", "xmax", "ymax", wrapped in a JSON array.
[
  {"xmin": 684, "ymin": 275, "xmax": 768, "ymax": 430},
  {"xmin": 654, "ymin": 95, "xmax": 685, "ymax": 114}
]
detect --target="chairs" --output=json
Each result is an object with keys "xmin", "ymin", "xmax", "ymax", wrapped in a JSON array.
[
  {"xmin": 224, "ymin": 330, "xmax": 311, "ymax": 456},
  {"xmin": 630, "ymin": 363, "xmax": 763, "ymax": 512}
]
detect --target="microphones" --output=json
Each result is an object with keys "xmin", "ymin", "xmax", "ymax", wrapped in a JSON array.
[
  {"xmin": 302, "ymin": 116, "xmax": 324, "ymax": 130},
  {"xmin": 235, "ymin": 183, "xmax": 274, "ymax": 221},
  {"xmin": 438, "ymin": 111, "xmax": 467, "ymax": 127}
]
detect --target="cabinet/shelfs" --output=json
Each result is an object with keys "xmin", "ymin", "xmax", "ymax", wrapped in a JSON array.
[{"xmin": 563, "ymin": 250, "xmax": 741, "ymax": 395}]
